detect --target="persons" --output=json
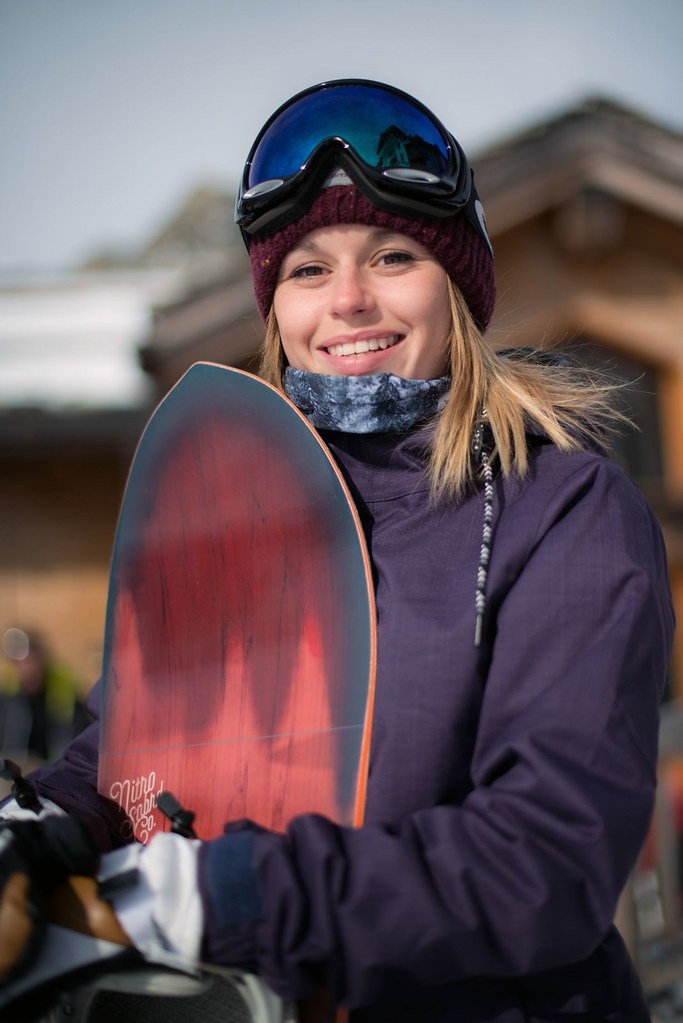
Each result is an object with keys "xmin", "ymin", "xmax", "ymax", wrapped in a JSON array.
[{"xmin": 2, "ymin": 79, "xmax": 679, "ymax": 1021}]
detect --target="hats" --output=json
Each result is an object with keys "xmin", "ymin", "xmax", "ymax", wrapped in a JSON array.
[{"xmin": 248, "ymin": 166, "xmax": 499, "ymax": 335}]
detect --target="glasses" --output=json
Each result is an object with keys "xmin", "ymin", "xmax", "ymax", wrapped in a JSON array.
[{"xmin": 233, "ymin": 79, "xmax": 495, "ymax": 259}]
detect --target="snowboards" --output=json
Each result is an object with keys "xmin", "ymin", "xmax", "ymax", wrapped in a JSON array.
[{"xmin": 97, "ymin": 358, "xmax": 378, "ymax": 1023}]
